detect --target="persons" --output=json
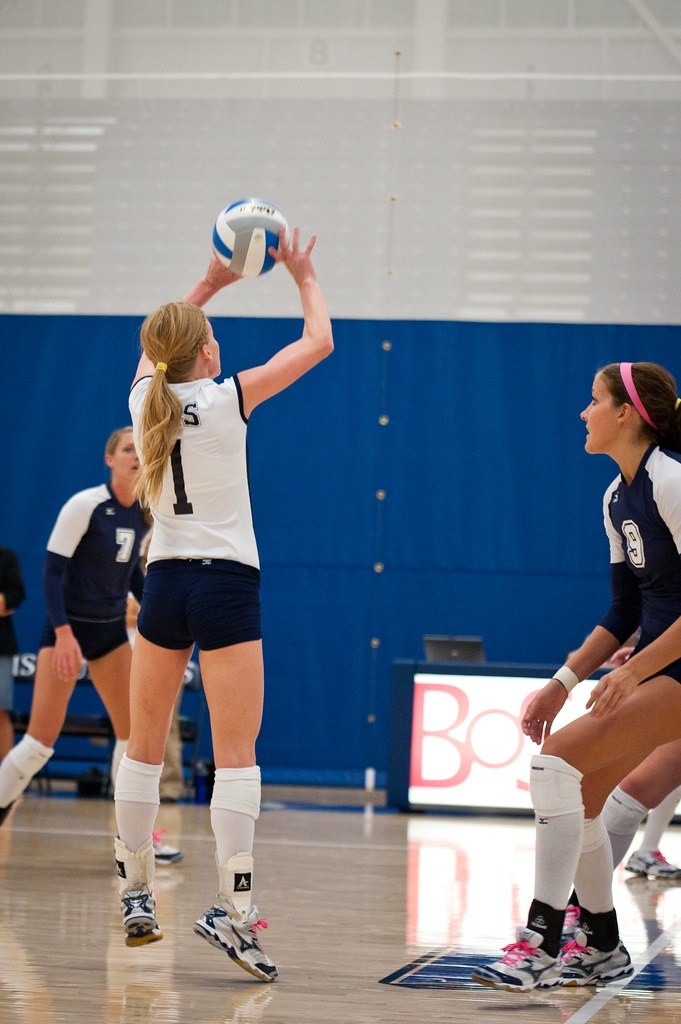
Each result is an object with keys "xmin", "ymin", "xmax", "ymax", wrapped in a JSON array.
[
  {"xmin": 0, "ymin": 545, "xmax": 26, "ymax": 831},
  {"xmin": 469, "ymin": 359, "xmax": 681, "ymax": 994},
  {"xmin": 0, "ymin": 424, "xmax": 186, "ymax": 869},
  {"xmin": 121, "ymin": 513, "xmax": 187, "ymax": 803},
  {"xmin": 113, "ymin": 225, "xmax": 338, "ymax": 985}
]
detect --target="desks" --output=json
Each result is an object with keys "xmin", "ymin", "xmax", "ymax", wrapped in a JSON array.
[
  {"xmin": 389, "ymin": 659, "xmax": 614, "ymax": 823},
  {"xmin": 405, "ymin": 816, "xmax": 681, "ymax": 959}
]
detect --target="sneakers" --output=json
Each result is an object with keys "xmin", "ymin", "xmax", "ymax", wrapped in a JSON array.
[
  {"xmin": 193, "ymin": 902, "xmax": 278, "ymax": 982},
  {"xmin": 624, "ymin": 849, "xmax": 681, "ymax": 879},
  {"xmin": 560, "ymin": 903, "xmax": 581, "ymax": 948},
  {"xmin": 121, "ymin": 891, "xmax": 162, "ymax": 947},
  {"xmin": 471, "ymin": 927, "xmax": 634, "ymax": 992},
  {"xmin": 153, "ymin": 832, "xmax": 184, "ymax": 865}
]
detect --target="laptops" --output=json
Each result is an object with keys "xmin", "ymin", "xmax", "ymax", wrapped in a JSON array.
[{"xmin": 425, "ymin": 636, "xmax": 485, "ymax": 662}]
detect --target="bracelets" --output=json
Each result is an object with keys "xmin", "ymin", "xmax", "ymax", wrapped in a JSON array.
[{"xmin": 552, "ymin": 665, "xmax": 580, "ymax": 694}]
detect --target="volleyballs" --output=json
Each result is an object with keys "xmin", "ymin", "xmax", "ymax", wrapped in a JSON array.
[{"xmin": 212, "ymin": 197, "xmax": 290, "ymax": 277}]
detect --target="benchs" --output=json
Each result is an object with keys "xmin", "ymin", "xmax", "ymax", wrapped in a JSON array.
[{"xmin": 9, "ymin": 713, "xmax": 197, "ymax": 796}]
